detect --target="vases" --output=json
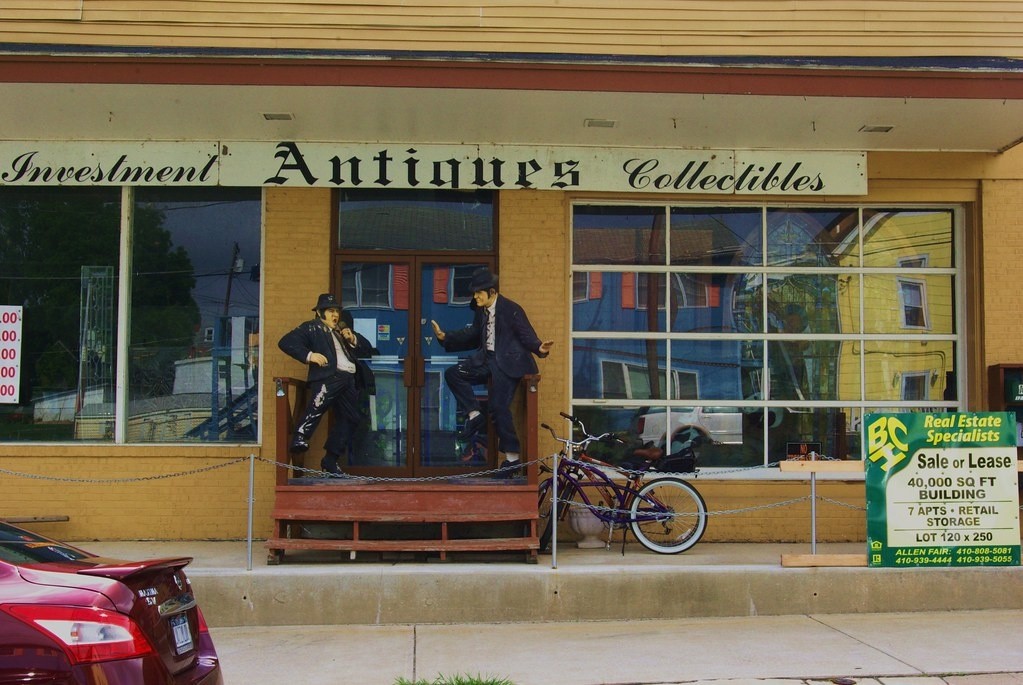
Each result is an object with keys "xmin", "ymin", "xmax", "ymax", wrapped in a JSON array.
[{"xmin": 568, "ymin": 507, "xmax": 607, "ymax": 549}]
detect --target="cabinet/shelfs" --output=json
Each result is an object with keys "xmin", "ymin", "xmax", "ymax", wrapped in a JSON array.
[{"xmin": 989, "ymin": 363, "xmax": 1023, "ymax": 460}]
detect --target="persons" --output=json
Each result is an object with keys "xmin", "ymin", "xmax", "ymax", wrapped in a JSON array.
[
  {"xmin": 431, "ymin": 270, "xmax": 554, "ymax": 479},
  {"xmin": 278, "ymin": 294, "xmax": 373, "ymax": 479}
]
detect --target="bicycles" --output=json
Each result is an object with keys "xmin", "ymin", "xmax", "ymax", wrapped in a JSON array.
[{"xmin": 537, "ymin": 412, "xmax": 708, "ymax": 556}]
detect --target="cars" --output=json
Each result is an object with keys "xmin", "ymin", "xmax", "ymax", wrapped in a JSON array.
[
  {"xmin": 634, "ymin": 395, "xmax": 743, "ymax": 450},
  {"xmin": 0, "ymin": 521, "xmax": 225, "ymax": 685}
]
